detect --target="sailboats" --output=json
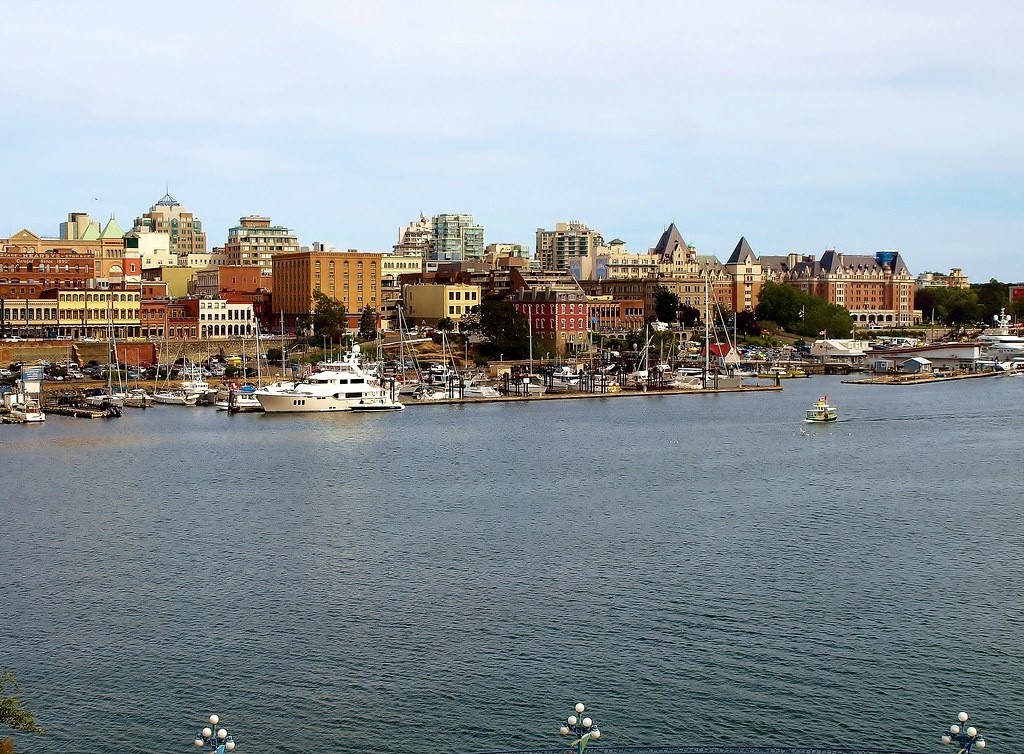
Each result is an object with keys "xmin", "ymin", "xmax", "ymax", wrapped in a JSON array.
[{"xmin": 0, "ymin": 272, "xmax": 742, "ymax": 425}]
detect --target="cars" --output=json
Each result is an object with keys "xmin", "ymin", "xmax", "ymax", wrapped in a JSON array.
[{"xmin": 744, "ymin": 344, "xmax": 819, "ymax": 358}]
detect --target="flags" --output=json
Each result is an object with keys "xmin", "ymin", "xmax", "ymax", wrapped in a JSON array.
[
  {"xmin": 850, "ymin": 330, "xmax": 854, "ymax": 334},
  {"xmin": 819, "ymin": 330, "xmax": 826, "ymax": 336}
]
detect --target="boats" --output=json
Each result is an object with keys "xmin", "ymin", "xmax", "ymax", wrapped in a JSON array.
[
  {"xmin": 804, "ymin": 395, "xmax": 838, "ymax": 425},
  {"xmin": 719, "ymin": 360, "xmax": 759, "ymax": 377},
  {"xmin": 1009, "ymin": 371, "xmax": 1023, "ymax": 377},
  {"xmin": 756, "ymin": 359, "xmax": 810, "ymax": 377},
  {"xmin": 253, "ymin": 335, "xmax": 406, "ymax": 414}
]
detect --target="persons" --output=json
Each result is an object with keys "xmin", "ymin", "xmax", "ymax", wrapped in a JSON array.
[
  {"xmin": 824, "ymin": 409, "xmax": 829, "ymax": 421},
  {"xmin": 236, "ymin": 382, "xmax": 243, "ymax": 389}
]
